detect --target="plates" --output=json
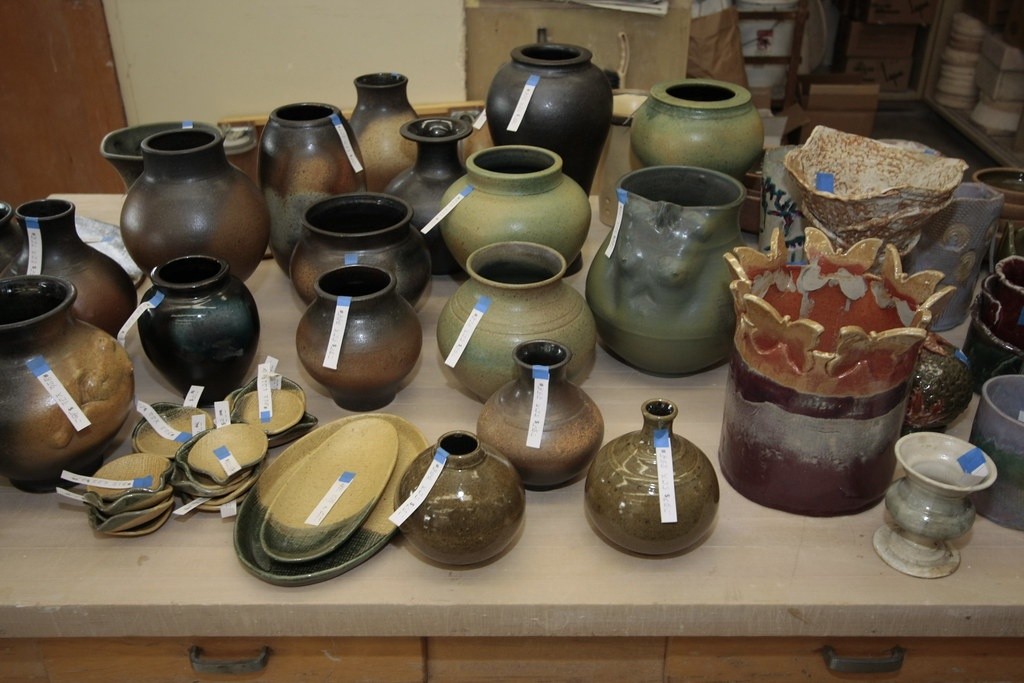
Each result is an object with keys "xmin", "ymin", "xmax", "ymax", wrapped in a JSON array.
[{"xmin": 82, "ymin": 372, "xmax": 433, "ymax": 588}]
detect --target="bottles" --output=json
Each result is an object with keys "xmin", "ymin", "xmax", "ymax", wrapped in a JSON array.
[
  {"xmin": 394, "ymin": 428, "xmax": 527, "ymax": 569},
  {"xmin": 436, "ymin": 241, "xmax": 597, "ymax": 406},
  {"xmin": 440, "ymin": 143, "xmax": 592, "ymax": 279},
  {"xmin": 256, "ymin": 97, "xmax": 368, "ymax": 280},
  {"xmin": 118, "ymin": 127, "xmax": 271, "ymax": 282},
  {"xmin": 135, "ymin": 254, "xmax": 261, "ymax": 410},
  {"xmin": 629, "ymin": 75, "xmax": 766, "ymax": 181},
  {"xmin": 584, "ymin": 394, "xmax": 720, "ymax": 558},
  {"xmin": 0, "ymin": 200, "xmax": 24, "ymax": 275},
  {"xmin": 295, "ymin": 264, "xmax": 424, "ymax": 414},
  {"xmin": 349, "ymin": 71, "xmax": 421, "ymax": 193},
  {"xmin": 485, "ymin": 39, "xmax": 614, "ymax": 202},
  {"xmin": 0, "ymin": 274, "xmax": 136, "ymax": 495},
  {"xmin": 290, "ymin": 190, "xmax": 434, "ymax": 308},
  {"xmin": 0, "ymin": 198, "xmax": 138, "ymax": 340},
  {"xmin": 381, "ymin": 115, "xmax": 475, "ymax": 278},
  {"xmin": 476, "ymin": 336, "xmax": 606, "ymax": 491}
]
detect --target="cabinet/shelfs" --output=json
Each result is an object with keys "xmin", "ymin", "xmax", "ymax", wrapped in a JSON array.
[
  {"xmin": 663, "ymin": 636, "xmax": 1024, "ymax": 683},
  {"xmin": 0, "ymin": 637, "xmax": 429, "ymax": 683}
]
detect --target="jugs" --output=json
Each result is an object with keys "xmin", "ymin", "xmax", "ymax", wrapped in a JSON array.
[{"xmin": 581, "ymin": 163, "xmax": 756, "ymax": 381}]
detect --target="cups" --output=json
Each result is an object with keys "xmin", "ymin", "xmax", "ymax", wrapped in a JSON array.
[{"xmin": 968, "ymin": 374, "xmax": 1024, "ymax": 533}]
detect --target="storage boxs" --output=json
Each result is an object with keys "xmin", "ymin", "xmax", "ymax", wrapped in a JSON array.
[
  {"xmin": 778, "ymin": 0, "xmax": 936, "ymax": 144},
  {"xmin": 974, "ymin": 55, "xmax": 1024, "ymax": 102},
  {"xmin": 979, "ymin": 32, "xmax": 1024, "ymax": 70}
]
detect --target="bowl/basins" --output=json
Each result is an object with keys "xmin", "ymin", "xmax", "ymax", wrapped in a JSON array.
[{"xmin": 971, "ymin": 167, "xmax": 1024, "ymax": 242}]
